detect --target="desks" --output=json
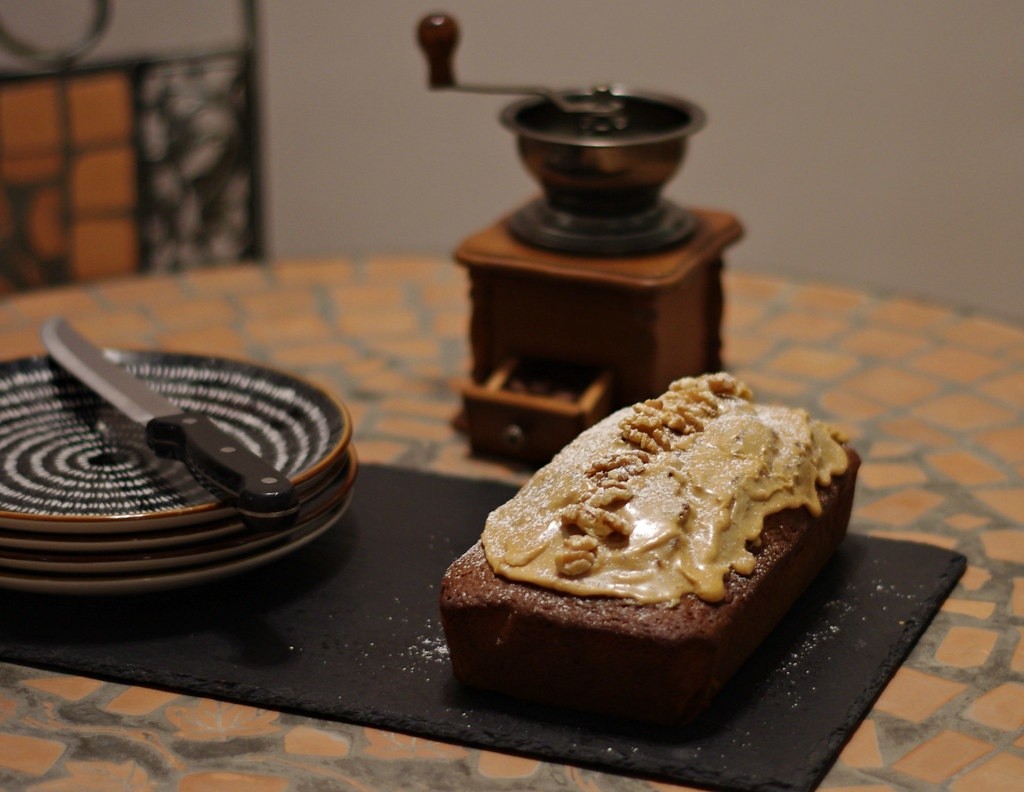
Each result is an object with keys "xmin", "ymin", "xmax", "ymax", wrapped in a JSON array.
[{"xmin": 0, "ymin": 252, "xmax": 1024, "ymax": 792}]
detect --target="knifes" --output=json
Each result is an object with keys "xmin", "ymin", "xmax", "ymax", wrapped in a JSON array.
[{"xmin": 41, "ymin": 311, "xmax": 299, "ymax": 531}]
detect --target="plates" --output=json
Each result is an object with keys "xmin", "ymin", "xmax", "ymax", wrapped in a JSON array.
[{"xmin": 0, "ymin": 344, "xmax": 355, "ymax": 619}]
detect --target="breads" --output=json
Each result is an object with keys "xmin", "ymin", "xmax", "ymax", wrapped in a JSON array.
[{"xmin": 441, "ymin": 366, "xmax": 863, "ymax": 715}]
